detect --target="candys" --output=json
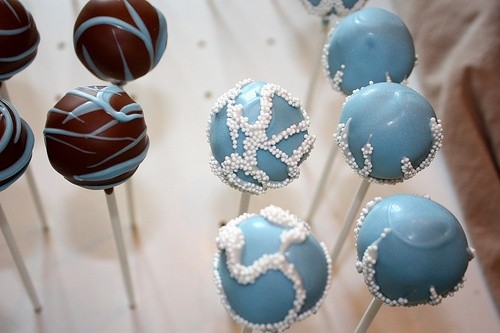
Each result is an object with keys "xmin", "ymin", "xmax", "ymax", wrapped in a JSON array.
[{"xmin": 0, "ymin": 2, "xmax": 475, "ymax": 333}]
